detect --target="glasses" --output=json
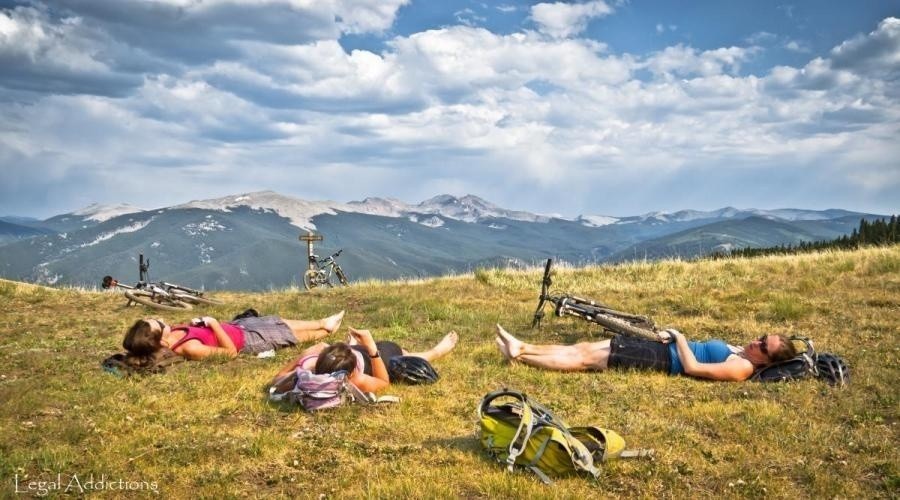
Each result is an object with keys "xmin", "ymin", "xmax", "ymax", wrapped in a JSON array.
[
  {"xmin": 148, "ymin": 318, "xmax": 165, "ymax": 336},
  {"xmin": 758, "ymin": 334, "xmax": 770, "ymax": 361}
]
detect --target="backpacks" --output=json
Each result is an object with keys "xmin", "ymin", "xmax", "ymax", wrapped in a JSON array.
[
  {"xmin": 267, "ymin": 366, "xmax": 399, "ymax": 414},
  {"xmin": 475, "ymin": 387, "xmax": 626, "ymax": 485},
  {"xmin": 751, "ymin": 334, "xmax": 821, "ymax": 384},
  {"xmin": 100, "ymin": 348, "xmax": 185, "ymax": 381}
]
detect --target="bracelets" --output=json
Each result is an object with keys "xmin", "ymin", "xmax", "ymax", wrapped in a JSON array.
[{"xmin": 368, "ymin": 350, "xmax": 380, "ymax": 358}]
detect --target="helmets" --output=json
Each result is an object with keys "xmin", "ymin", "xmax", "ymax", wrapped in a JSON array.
[
  {"xmin": 816, "ymin": 351, "xmax": 856, "ymax": 387},
  {"xmin": 387, "ymin": 355, "xmax": 440, "ymax": 386}
]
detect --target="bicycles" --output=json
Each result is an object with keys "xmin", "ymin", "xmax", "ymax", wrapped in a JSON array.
[
  {"xmin": 102, "ymin": 252, "xmax": 226, "ymax": 313},
  {"xmin": 302, "ymin": 249, "xmax": 351, "ymax": 293},
  {"xmin": 531, "ymin": 258, "xmax": 661, "ymax": 342}
]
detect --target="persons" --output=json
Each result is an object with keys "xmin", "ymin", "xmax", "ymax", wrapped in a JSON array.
[
  {"xmin": 123, "ymin": 309, "xmax": 346, "ymax": 372},
  {"xmin": 271, "ymin": 326, "xmax": 458, "ymax": 393},
  {"xmin": 496, "ymin": 322, "xmax": 796, "ymax": 382}
]
